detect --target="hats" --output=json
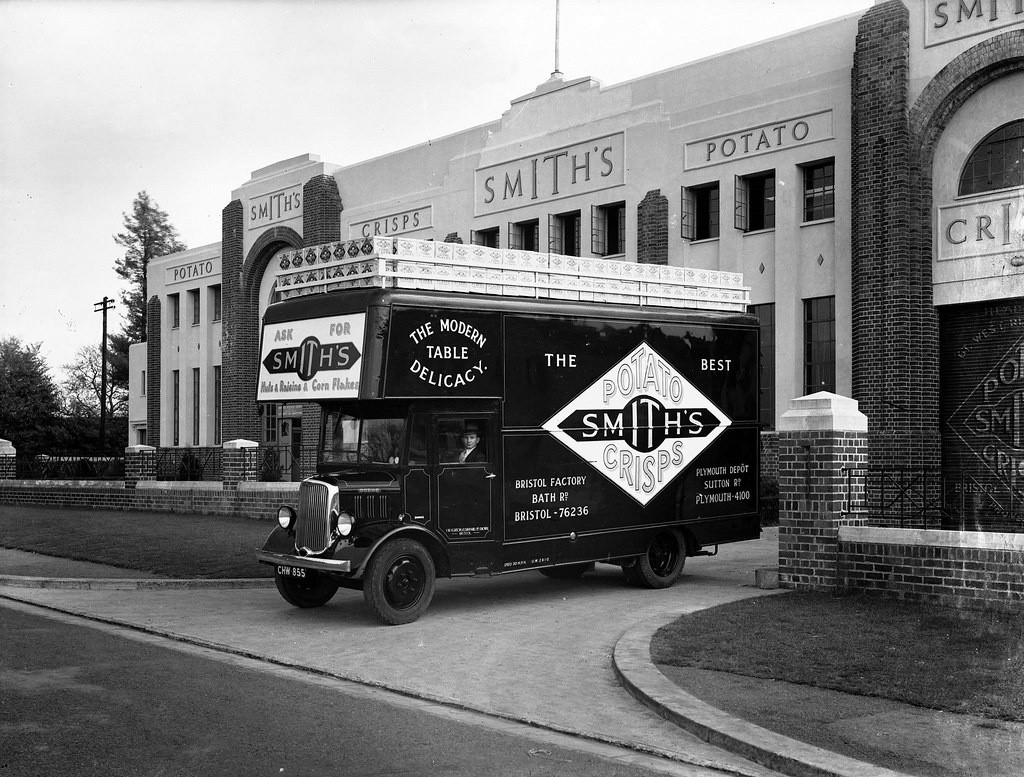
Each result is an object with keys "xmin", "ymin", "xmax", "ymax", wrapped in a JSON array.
[{"xmin": 462, "ymin": 426, "xmax": 478, "ymax": 435}]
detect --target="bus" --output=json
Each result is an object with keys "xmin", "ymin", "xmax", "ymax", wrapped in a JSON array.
[{"xmin": 257, "ymin": 237, "xmax": 762, "ymax": 625}]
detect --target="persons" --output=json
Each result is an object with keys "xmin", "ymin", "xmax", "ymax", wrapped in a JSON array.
[{"xmin": 446, "ymin": 424, "xmax": 486, "ymax": 462}]
